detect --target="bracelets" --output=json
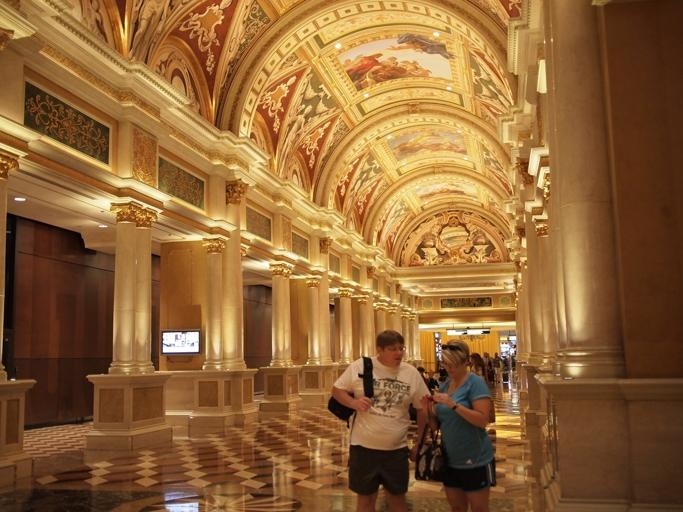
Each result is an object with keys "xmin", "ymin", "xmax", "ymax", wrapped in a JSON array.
[{"xmin": 451, "ymin": 402, "xmax": 460, "ymax": 409}]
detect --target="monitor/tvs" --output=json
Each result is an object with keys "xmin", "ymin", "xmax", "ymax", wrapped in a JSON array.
[{"xmin": 158, "ymin": 329, "xmax": 200, "ymax": 355}]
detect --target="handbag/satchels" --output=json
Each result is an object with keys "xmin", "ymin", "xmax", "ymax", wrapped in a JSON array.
[
  {"xmin": 415, "ymin": 444, "xmax": 445, "ymax": 481},
  {"xmin": 328, "ymin": 357, "xmax": 375, "ymax": 420}
]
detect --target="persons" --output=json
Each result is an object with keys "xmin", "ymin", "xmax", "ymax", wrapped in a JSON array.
[
  {"xmin": 419, "ymin": 337, "xmax": 496, "ymax": 511},
  {"xmin": 416, "ymin": 348, "xmax": 517, "ymax": 396},
  {"xmin": 328, "ymin": 327, "xmax": 431, "ymax": 511}
]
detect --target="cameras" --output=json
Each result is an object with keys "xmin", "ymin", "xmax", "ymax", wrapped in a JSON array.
[{"xmin": 425, "ymin": 396, "xmax": 437, "ymax": 404}]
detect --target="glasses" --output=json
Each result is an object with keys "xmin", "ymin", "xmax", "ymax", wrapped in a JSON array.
[
  {"xmin": 442, "ymin": 344, "xmax": 459, "ymax": 350},
  {"xmin": 387, "ymin": 345, "xmax": 406, "ymax": 351}
]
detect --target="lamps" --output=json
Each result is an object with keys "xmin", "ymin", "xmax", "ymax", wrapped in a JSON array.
[
  {"xmin": 514, "ymin": 175, "xmax": 552, "ymax": 311},
  {"xmin": 202, "ymin": 237, "xmax": 417, "ymax": 321},
  {"xmin": 110, "ymin": 201, "xmax": 158, "ymax": 228}
]
